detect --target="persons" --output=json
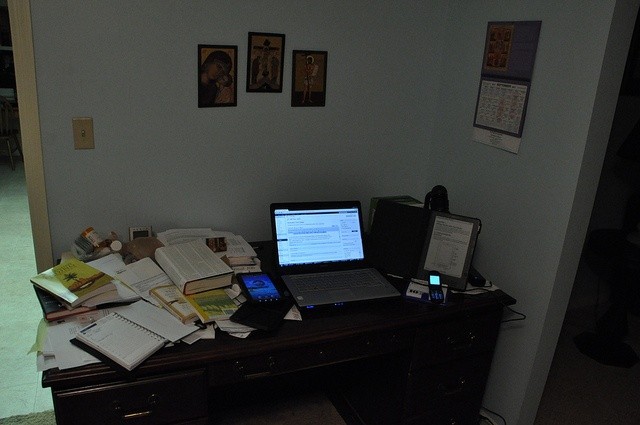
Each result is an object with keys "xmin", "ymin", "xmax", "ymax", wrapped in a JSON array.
[
  {"xmin": 270, "ymin": 56, "xmax": 279, "ymax": 84},
  {"xmin": 252, "ymin": 56, "xmax": 261, "ymax": 84},
  {"xmin": 302, "ymin": 55, "xmax": 318, "ymax": 104},
  {"xmin": 201, "ymin": 50, "xmax": 232, "ymax": 103},
  {"xmin": 215, "ymin": 73, "xmax": 232, "ymax": 103}
]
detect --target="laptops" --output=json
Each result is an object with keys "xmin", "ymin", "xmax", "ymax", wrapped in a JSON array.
[{"xmin": 269, "ymin": 200, "xmax": 401, "ymax": 308}]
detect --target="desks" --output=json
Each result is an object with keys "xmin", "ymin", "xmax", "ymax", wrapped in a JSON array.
[{"xmin": 42, "ymin": 240, "xmax": 518, "ymax": 425}]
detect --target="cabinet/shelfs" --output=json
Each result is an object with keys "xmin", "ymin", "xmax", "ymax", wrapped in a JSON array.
[
  {"xmin": 318, "ymin": 308, "xmax": 503, "ymax": 425},
  {"xmin": 52, "ymin": 366, "xmax": 211, "ymax": 425},
  {"xmin": 206, "ymin": 322, "xmax": 417, "ymax": 388}
]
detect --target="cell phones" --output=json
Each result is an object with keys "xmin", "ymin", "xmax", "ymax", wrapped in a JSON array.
[
  {"xmin": 428, "ymin": 270, "xmax": 444, "ymax": 303},
  {"xmin": 236, "ymin": 271, "xmax": 284, "ymax": 307}
]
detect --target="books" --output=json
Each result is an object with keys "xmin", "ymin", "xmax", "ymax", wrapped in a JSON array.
[{"xmin": 30, "ymin": 228, "xmax": 263, "ymax": 372}]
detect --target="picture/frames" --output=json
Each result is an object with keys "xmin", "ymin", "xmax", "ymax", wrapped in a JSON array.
[
  {"xmin": 246, "ymin": 31, "xmax": 286, "ymax": 94},
  {"xmin": 290, "ymin": 48, "xmax": 327, "ymax": 108},
  {"xmin": 196, "ymin": 43, "xmax": 238, "ymax": 109}
]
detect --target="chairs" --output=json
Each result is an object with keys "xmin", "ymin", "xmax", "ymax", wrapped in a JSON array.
[{"xmin": 0, "ymin": 98, "xmax": 23, "ymax": 170}]
